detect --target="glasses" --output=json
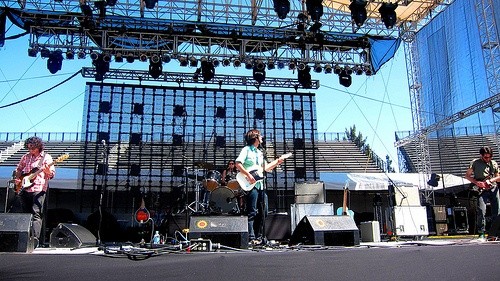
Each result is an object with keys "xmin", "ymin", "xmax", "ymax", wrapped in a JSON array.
[
  {"xmin": 28, "ymin": 146, "xmax": 37, "ymax": 151},
  {"xmin": 483, "ymin": 155, "xmax": 492, "ymax": 159},
  {"xmin": 229, "ymin": 163, "xmax": 234, "ymax": 165}
]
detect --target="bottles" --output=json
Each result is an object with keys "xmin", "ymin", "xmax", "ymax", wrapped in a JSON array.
[{"xmin": 154, "ymin": 231, "xmax": 160, "ymax": 244}]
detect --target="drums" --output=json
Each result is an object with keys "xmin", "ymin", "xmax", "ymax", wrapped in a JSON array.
[
  {"xmin": 208, "ymin": 185, "xmax": 237, "ymax": 214},
  {"xmin": 194, "ymin": 162, "xmax": 218, "ymax": 169},
  {"xmin": 203, "ymin": 170, "xmax": 223, "ymax": 192},
  {"xmin": 227, "ymin": 172, "xmax": 242, "ymax": 192},
  {"xmin": 238, "ymin": 194, "xmax": 246, "ymax": 209}
]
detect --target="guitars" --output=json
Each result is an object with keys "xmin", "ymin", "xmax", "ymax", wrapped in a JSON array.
[
  {"xmin": 236, "ymin": 152, "xmax": 292, "ymax": 191},
  {"xmin": 468, "ymin": 176, "xmax": 500, "ymax": 197},
  {"xmin": 17, "ymin": 155, "xmax": 69, "ymax": 189}
]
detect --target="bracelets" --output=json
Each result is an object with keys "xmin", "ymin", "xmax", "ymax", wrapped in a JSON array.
[{"xmin": 47, "ymin": 172, "xmax": 53, "ymax": 176}]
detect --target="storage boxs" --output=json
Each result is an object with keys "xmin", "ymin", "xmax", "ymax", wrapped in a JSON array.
[{"xmin": 385, "ymin": 207, "xmax": 429, "ymax": 240}]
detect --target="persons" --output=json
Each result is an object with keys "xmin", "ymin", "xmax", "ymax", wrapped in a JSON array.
[
  {"xmin": 234, "ymin": 130, "xmax": 283, "ymax": 247},
  {"xmin": 222, "ymin": 161, "xmax": 244, "ymax": 210},
  {"xmin": 466, "ymin": 146, "xmax": 500, "ymax": 240},
  {"xmin": 12, "ymin": 137, "xmax": 55, "ymax": 247}
]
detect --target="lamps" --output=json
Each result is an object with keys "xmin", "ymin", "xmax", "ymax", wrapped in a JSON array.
[
  {"xmin": 298, "ymin": 70, "xmax": 312, "ymax": 89},
  {"xmin": 344, "ymin": 64, "xmax": 353, "ymax": 75},
  {"xmin": 353, "ymin": 65, "xmax": 363, "ymax": 75},
  {"xmin": 298, "ymin": 62, "xmax": 311, "ymax": 73},
  {"xmin": 273, "ymin": 0, "xmax": 290, "ymax": 19},
  {"xmin": 340, "ymin": 73, "xmax": 352, "ymax": 87},
  {"xmin": 288, "ymin": 62, "xmax": 297, "ymax": 70},
  {"xmin": 313, "ymin": 62, "xmax": 322, "ymax": 73},
  {"xmin": 305, "ymin": 0, "xmax": 323, "ymax": 23},
  {"xmin": 349, "ymin": 0, "xmax": 367, "ymax": 25},
  {"xmin": 277, "ymin": 61, "xmax": 285, "ymax": 69},
  {"xmin": 324, "ymin": 63, "xmax": 332, "ymax": 74},
  {"xmin": 28, "ymin": 46, "xmax": 275, "ymax": 82},
  {"xmin": 364, "ymin": 67, "xmax": 372, "ymax": 76},
  {"xmin": 334, "ymin": 64, "xmax": 343, "ymax": 74},
  {"xmin": 380, "ymin": 3, "xmax": 397, "ymax": 29}
]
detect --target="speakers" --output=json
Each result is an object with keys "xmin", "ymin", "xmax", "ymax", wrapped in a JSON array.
[
  {"xmin": 451, "ymin": 207, "xmax": 469, "ymax": 233},
  {"xmin": 50, "ymin": 223, "xmax": 97, "ymax": 248},
  {"xmin": 294, "ymin": 181, "xmax": 326, "ymax": 204},
  {"xmin": 389, "ymin": 185, "xmax": 421, "ymax": 206},
  {"xmin": 361, "ymin": 221, "xmax": 380, "ymax": 242},
  {"xmin": 0, "ymin": 213, "xmax": 34, "ymax": 253},
  {"xmin": 259, "ymin": 213, "xmax": 291, "ymax": 239},
  {"xmin": 189, "ymin": 216, "xmax": 248, "ymax": 248},
  {"xmin": 288, "ymin": 214, "xmax": 359, "ymax": 247}
]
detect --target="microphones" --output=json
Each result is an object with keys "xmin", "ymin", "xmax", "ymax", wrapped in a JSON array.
[{"xmin": 101, "ymin": 139, "xmax": 105, "ymax": 145}]
